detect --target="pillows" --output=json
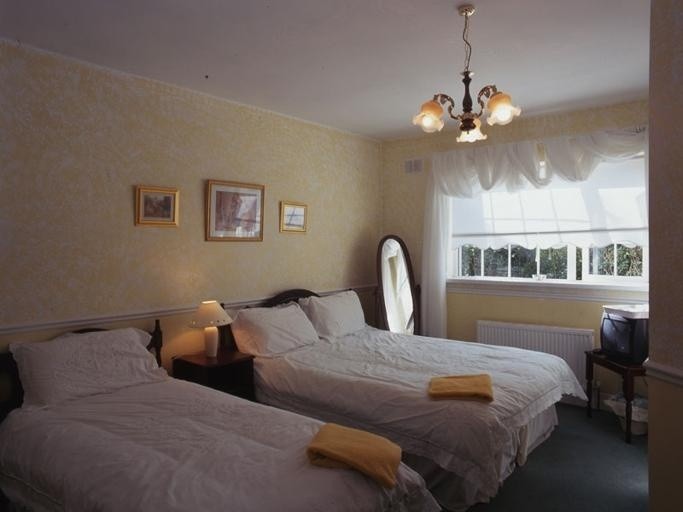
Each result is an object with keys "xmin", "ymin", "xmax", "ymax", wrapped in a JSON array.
[
  {"xmin": 6, "ymin": 326, "xmax": 170, "ymax": 409},
  {"xmin": 299, "ymin": 287, "xmax": 367, "ymax": 342},
  {"xmin": 229, "ymin": 303, "xmax": 320, "ymax": 356}
]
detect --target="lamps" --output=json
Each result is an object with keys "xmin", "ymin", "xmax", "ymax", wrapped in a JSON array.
[
  {"xmin": 188, "ymin": 299, "xmax": 237, "ymax": 357},
  {"xmin": 412, "ymin": 1, "xmax": 522, "ymax": 145}
]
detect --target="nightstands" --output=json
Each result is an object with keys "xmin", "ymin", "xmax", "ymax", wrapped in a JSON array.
[{"xmin": 170, "ymin": 352, "xmax": 257, "ymax": 405}]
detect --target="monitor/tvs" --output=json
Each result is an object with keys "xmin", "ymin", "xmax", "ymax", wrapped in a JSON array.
[{"xmin": 600, "ymin": 312, "xmax": 649, "ymax": 367}]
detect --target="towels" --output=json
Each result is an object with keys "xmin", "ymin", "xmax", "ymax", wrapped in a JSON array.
[
  {"xmin": 426, "ymin": 372, "xmax": 494, "ymax": 403},
  {"xmin": 304, "ymin": 421, "xmax": 402, "ymax": 489}
]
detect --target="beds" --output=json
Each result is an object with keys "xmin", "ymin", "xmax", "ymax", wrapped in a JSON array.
[
  {"xmin": 218, "ymin": 287, "xmax": 590, "ymax": 512},
  {"xmin": 0, "ymin": 319, "xmax": 450, "ymax": 512}
]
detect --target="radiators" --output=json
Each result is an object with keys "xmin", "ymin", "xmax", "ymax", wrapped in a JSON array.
[{"xmin": 474, "ymin": 319, "xmax": 596, "ymax": 413}]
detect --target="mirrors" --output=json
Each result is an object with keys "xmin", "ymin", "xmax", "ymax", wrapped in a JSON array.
[{"xmin": 375, "ymin": 234, "xmax": 420, "ymax": 335}]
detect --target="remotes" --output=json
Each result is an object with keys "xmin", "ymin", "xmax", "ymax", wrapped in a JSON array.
[{"xmin": 593, "ymin": 349, "xmax": 602, "ymax": 354}]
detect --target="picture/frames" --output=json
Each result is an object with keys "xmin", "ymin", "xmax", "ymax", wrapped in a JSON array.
[
  {"xmin": 204, "ymin": 179, "xmax": 266, "ymax": 244},
  {"xmin": 278, "ymin": 199, "xmax": 309, "ymax": 235},
  {"xmin": 131, "ymin": 183, "xmax": 181, "ymax": 227}
]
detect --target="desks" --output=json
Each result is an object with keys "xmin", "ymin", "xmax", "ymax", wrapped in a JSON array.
[{"xmin": 583, "ymin": 348, "xmax": 649, "ymax": 445}]
